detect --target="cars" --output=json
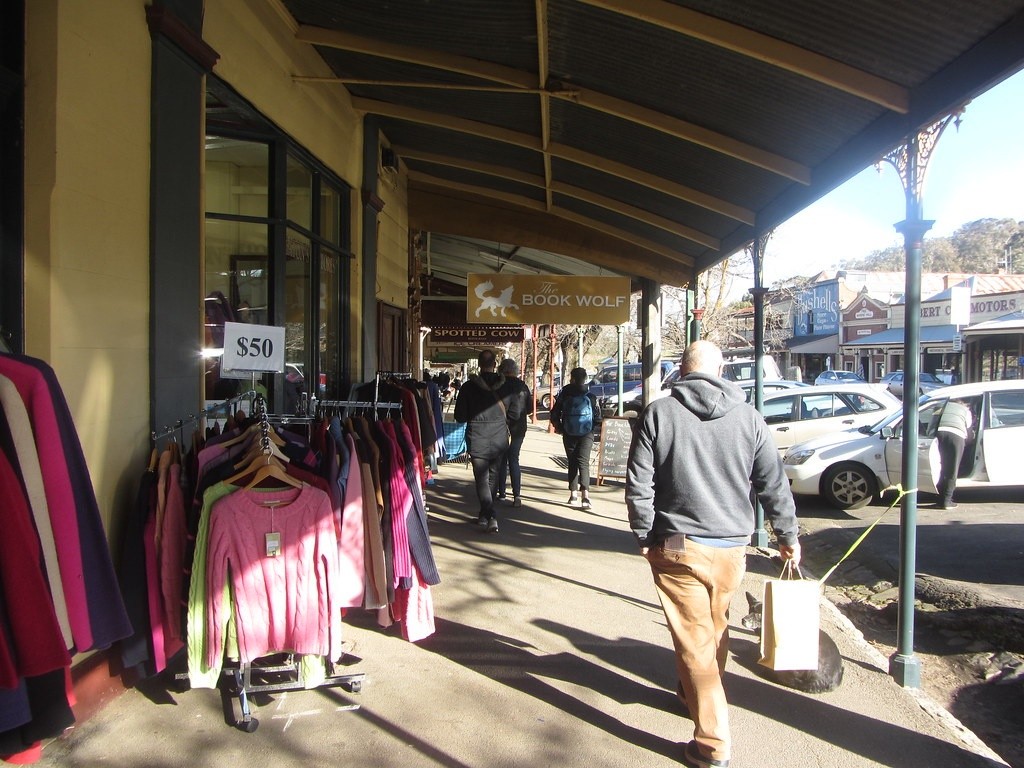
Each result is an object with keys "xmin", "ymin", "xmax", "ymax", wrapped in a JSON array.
[
  {"xmin": 531, "ymin": 377, "xmax": 561, "ymax": 409},
  {"xmin": 601, "ymin": 354, "xmax": 1024, "ymax": 511}
]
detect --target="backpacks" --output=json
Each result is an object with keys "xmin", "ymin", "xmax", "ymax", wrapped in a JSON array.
[{"xmin": 563, "ymin": 389, "xmax": 593, "ymax": 437}]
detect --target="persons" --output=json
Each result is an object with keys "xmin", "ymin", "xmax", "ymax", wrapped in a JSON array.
[
  {"xmin": 623, "ymin": 339, "xmax": 800, "ymax": 765},
  {"xmin": 934, "ymin": 399, "xmax": 976, "ymax": 509},
  {"xmin": 423, "ymin": 351, "xmax": 535, "ymax": 532},
  {"xmin": 550, "ymin": 368, "xmax": 602, "ymax": 507}
]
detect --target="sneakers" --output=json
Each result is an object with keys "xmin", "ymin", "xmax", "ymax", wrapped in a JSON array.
[
  {"xmin": 685, "ymin": 739, "xmax": 729, "ymax": 768},
  {"xmin": 677, "ymin": 679, "xmax": 688, "ymax": 706}
]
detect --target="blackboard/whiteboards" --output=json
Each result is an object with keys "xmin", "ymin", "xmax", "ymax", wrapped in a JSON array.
[{"xmin": 598, "ymin": 417, "xmax": 633, "ymax": 478}]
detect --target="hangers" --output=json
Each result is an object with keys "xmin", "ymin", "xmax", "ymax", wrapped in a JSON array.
[{"xmin": 147, "ymin": 372, "xmax": 424, "ymax": 495}]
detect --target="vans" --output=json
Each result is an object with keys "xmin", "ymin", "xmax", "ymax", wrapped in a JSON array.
[{"xmin": 586, "ymin": 361, "xmax": 675, "ymax": 417}]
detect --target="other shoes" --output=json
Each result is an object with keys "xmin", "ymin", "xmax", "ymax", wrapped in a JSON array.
[
  {"xmin": 499, "ymin": 494, "xmax": 505, "ymax": 500},
  {"xmin": 936, "ymin": 503, "xmax": 944, "ymax": 508},
  {"xmin": 478, "ymin": 514, "xmax": 488, "ymax": 526},
  {"xmin": 568, "ymin": 497, "xmax": 577, "ymax": 505},
  {"xmin": 513, "ymin": 496, "xmax": 521, "ymax": 507},
  {"xmin": 582, "ymin": 498, "xmax": 591, "ymax": 509},
  {"xmin": 943, "ymin": 504, "xmax": 958, "ymax": 510},
  {"xmin": 488, "ymin": 519, "xmax": 498, "ymax": 534}
]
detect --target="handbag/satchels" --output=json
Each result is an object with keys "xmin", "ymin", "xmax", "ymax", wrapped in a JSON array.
[{"xmin": 758, "ymin": 558, "xmax": 823, "ymax": 671}]
